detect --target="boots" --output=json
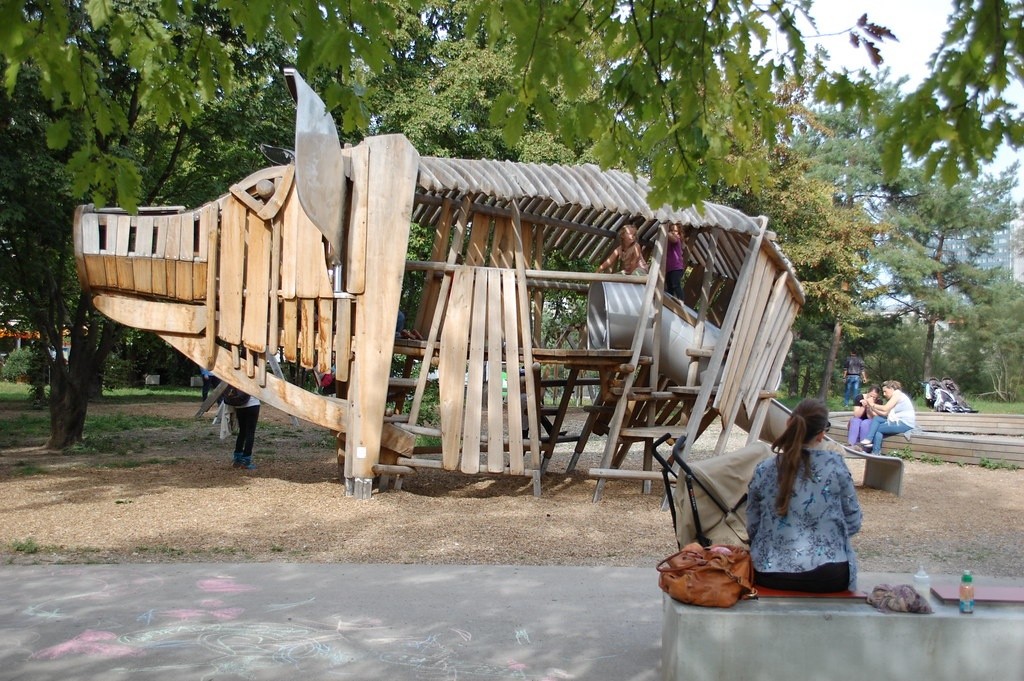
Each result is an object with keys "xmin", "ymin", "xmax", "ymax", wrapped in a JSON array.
[{"xmin": 232, "ymin": 451, "xmax": 257, "ymax": 469}]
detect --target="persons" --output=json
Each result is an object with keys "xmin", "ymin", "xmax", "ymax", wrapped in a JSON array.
[
  {"xmin": 848, "ymin": 386, "xmax": 883, "ymax": 453},
  {"xmin": 506, "ymin": 368, "xmax": 545, "ymax": 468},
  {"xmin": 861, "ymin": 381, "xmax": 915, "ymax": 456},
  {"xmin": 595, "ymin": 225, "xmax": 648, "ymax": 275},
  {"xmin": 665, "ymin": 223, "xmax": 684, "ymax": 305},
  {"xmin": 200, "ymin": 364, "xmax": 260, "ymax": 469},
  {"xmin": 313, "ymin": 351, "xmax": 337, "ymax": 394},
  {"xmin": 747, "ymin": 400, "xmax": 862, "ymax": 592},
  {"xmin": 842, "ymin": 350, "xmax": 866, "ymax": 407},
  {"xmin": 396, "ymin": 310, "xmax": 423, "ymax": 340}
]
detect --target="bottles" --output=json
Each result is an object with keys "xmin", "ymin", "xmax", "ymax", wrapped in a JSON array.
[
  {"xmin": 958, "ymin": 570, "xmax": 975, "ymax": 614},
  {"xmin": 912, "ymin": 564, "xmax": 931, "ymax": 604}
]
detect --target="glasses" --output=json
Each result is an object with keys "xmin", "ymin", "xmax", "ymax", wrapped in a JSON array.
[{"xmin": 821, "ymin": 422, "xmax": 832, "ymax": 431}]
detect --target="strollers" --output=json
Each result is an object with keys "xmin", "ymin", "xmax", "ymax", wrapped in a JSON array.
[{"xmin": 649, "ymin": 433, "xmax": 775, "ymax": 554}]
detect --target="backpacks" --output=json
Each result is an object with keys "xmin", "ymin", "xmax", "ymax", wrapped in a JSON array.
[{"xmin": 223, "ymin": 384, "xmax": 250, "ymax": 406}]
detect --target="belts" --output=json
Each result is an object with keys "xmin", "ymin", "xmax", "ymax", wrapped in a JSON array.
[{"xmin": 846, "ymin": 373, "xmax": 859, "ymax": 375}]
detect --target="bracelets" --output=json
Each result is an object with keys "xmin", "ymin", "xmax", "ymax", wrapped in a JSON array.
[
  {"xmin": 843, "ymin": 378, "xmax": 845, "ymax": 379},
  {"xmin": 873, "ymin": 403, "xmax": 875, "ymax": 407}
]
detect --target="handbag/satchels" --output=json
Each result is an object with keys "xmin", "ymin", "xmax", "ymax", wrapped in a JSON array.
[{"xmin": 656, "ymin": 541, "xmax": 760, "ymax": 608}]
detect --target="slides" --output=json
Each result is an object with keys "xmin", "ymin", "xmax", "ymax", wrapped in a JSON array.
[{"xmin": 586, "ymin": 278, "xmax": 906, "ymax": 498}]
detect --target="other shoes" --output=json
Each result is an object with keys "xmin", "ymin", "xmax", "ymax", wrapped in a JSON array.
[{"xmin": 854, "ymin": 442, "xmax": 874, "ymax": 448}]
